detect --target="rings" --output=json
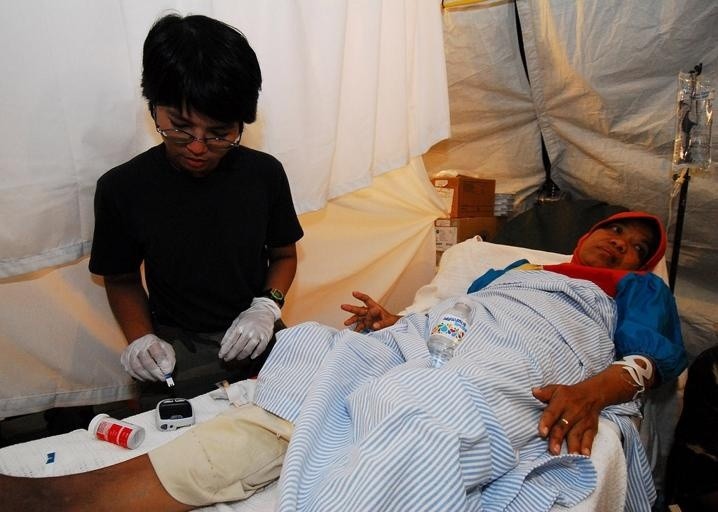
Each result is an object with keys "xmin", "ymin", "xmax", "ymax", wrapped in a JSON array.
[{"xmin": 562, "ymin": 418, "xmax": 572, "ymax": 428}]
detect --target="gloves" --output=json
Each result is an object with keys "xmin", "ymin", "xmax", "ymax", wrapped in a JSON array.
[
  {"xmin": 218, "ymin": 297, "xmax": 281, "ymax": 361},
  {"xmin": 120, "ymin": 334, "xmax": 175, "ymax": 382}
]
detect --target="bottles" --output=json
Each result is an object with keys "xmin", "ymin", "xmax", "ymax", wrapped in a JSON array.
[{"xmin": 426, "ymin": 301, "xmax": 472, "ymax": 368}]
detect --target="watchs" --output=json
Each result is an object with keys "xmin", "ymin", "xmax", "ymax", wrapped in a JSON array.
[{"xmin": 259, "ymin": 286, "xmax": 286, "ymax": 309}]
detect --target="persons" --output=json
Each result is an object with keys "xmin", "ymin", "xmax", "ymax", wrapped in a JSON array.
[{"xmin": 87, "ymin": 9, "xmax": 303, "ymax": 411}]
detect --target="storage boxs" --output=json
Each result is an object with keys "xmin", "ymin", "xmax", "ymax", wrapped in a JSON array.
[
  {"xmin": 434, "ymin": 218, "xmax": 498, "ymax": 267},
  {"xmin": 431, "ymin": 173, "xmax": 497, "ymax": 219}
]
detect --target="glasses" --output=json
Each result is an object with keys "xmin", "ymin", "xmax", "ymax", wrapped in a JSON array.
[{"xmin": 155, "ymin": 112, "xmax": 241, "ymax": 150}]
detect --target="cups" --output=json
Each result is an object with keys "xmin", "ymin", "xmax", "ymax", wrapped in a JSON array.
[{"xmin": 88, "ymin": 412, "xmax": 144, "ymax": 449}]
